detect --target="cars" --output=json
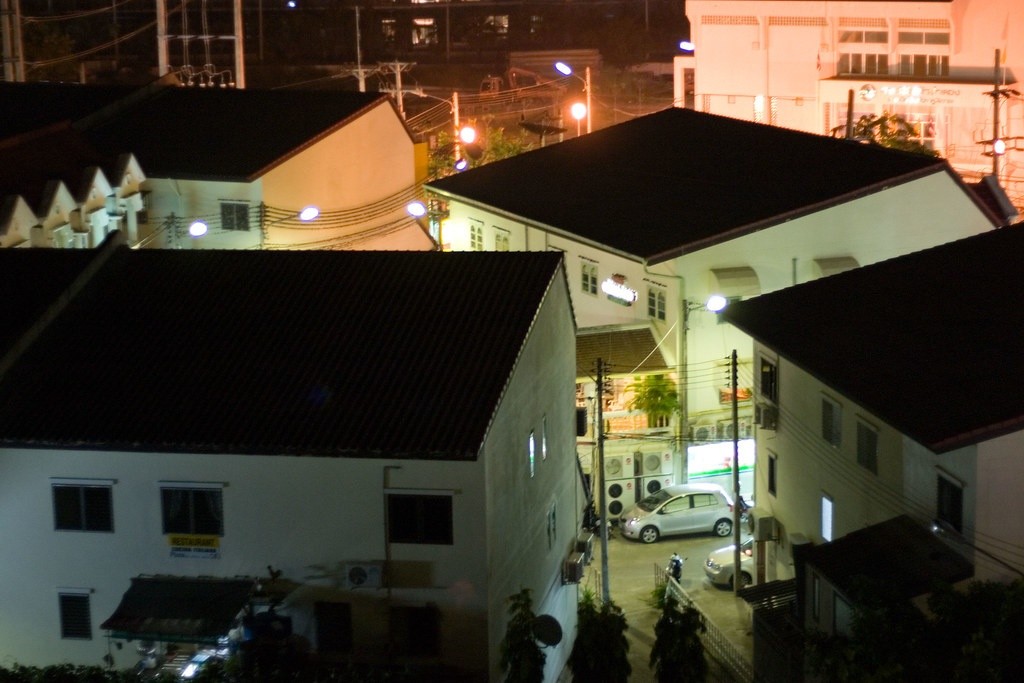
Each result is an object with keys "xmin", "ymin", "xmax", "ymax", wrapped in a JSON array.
[
  {"xmin": 619, "ymin": 479, "xmax": 733, "ymax": 541},
  {"xmin": 705, "ymin": 534, "xmax": 753, "ymax": 588}
]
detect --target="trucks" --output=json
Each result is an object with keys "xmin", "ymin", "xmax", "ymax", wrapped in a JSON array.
[{"xmin": 509, "ymin": 48, "xmax": 619, "ymax": 82}]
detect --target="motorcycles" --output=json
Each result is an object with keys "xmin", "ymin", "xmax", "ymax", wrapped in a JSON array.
[
  {"xmin": 664, "ymin": 550, "xmax": 689, "ymax": 609},
  {"xmin": 585, "ymin": 501, "xmax": 614, "ymax": 542}
]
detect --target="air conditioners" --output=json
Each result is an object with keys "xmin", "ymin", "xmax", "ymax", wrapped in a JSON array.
[
  {"xmin": 575, "ymin": 531, "xmax": 594, "ymax": 566},
  {"xmin": 690, "ymin": 424, "xmax": 717, "ymax": 444},
  {"xmin": 752, "ymin": 403, "xmax": 779, "ymax": 430},
  {"xmin": 747, "ymin": 506, "xmax": 780, "ymax": 546},
  {"xmin": 603, "ymin": 448, "xmax": 675, "ymax": 520},
  {"xmin": 562, "ymin": 551, "xmax": 585, "ymax": 585},
  {"xmin": 718, "ymin": 418, "xmax": 747, "ymax": 439},
  {"xmin": 345, "ymin": 562, "xmax": 388, "ymax": 590}
]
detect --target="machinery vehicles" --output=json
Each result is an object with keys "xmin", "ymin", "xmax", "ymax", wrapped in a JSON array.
[{"xmin": 479, "ymin": 66, "xmax": 567, "ymax": 108}]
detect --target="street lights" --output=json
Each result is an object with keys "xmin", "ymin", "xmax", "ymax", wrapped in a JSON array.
[
  {"xmin": 681, "ymin": 294, "xmax": 727, "ymax": 483},
  {"xmin": 571, "ymin": 101, "xmax": 587, "ymax": 137},
  {"xmin": 258, "ymin": 207, "xmax": 320, "ymax": 249},
  {"xmin": 556, "ymin": 61, "xmax": 590, "ymax": 134},
  {"xmin": 462, "ymin": 128, "xmax": 476, "ymax": 170},
  {"xmin": 410, "ymin": 90, "xmax": 460, "ymax": 172},
  {"xmin": 174, "ymin": 222, "xmax": 207, "ymax": 248},
  {"xmin": 407, "ymin": 204, "xmax": 442, "ymax": 253}
]
